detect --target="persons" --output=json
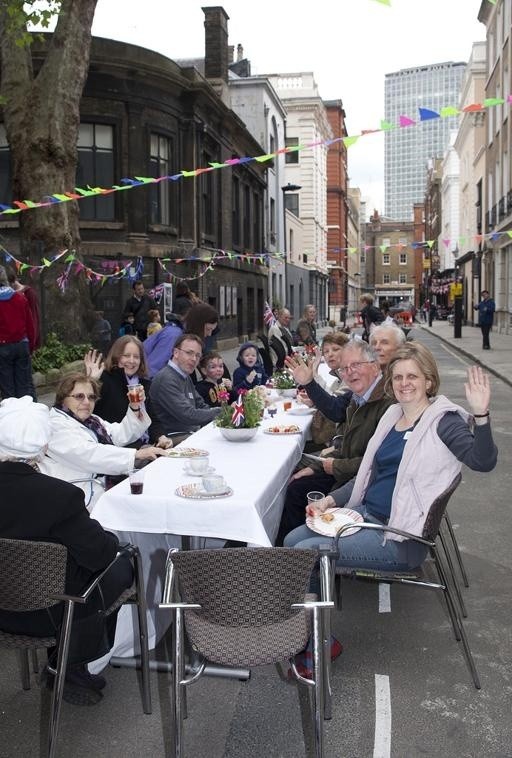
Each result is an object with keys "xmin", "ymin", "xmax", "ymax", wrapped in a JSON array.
[
  {"xmin": 37, "ymin": 302, "xmax": 233, "ymax": 515},
  {"xmin": 1, "ymin": 266, "xmax": 43, "ymax": 402},
  {"xmin": 471, "ymin": 289, "xmax": 495, "ymax": 352},
  {"xmin": 283, "ymin": 323, "xmax": 407, "ymax": 533},
  {"xmin": 282, "ymin": 340, "xmax": 499, "ymax": 679},
  {"xmin": 231, "ymin": 293, "xmax": 436, "ymax": 402},
  {"xmin": 89, "ymin": 279, "xmax": 222, "ymax": 365},
  {"xmin": 1, "ymin": 396, "xmax": 139, "ymax": 708}
]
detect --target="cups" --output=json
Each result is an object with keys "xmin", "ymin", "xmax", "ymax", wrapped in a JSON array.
[
  {"xmin": 283, "ymin": 397, "xmax": 292, "ymax": 410},
  {"xmin": 126, "ymin": 384, "xmax": 143, "ymax": 404},
  {"xmin": 307, "ymin": 491, "xmax": 326, "ymax": 519},
  {"xmin": 201, "ymin": 474, "xmax": 228, "ymax": 490},
  {"xmin": 127, "ymin": 469, "xmax": 145, "ymax": 493}
]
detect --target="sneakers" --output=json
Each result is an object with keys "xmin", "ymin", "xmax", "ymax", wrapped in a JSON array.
[{"xmin": 37, "ymin": 662, "xmax": 106, "ymax": 706}]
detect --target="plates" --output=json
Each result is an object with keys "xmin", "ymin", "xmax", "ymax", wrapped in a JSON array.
[
  {"xmin": 184, "ymin": 465, "xmax": 216, "ymax": 476},
  {"xmin": 288, "ymin": 407, "xmax": 317, "ymax": 416},
  {"xmin": 306, "ymin": 506, "xmax": 364, "ymax": 537},
  {"xmin": 165, "ymin": 446, "xmax": 209, "ymax": 458},
  {"xmin": 175, "ymin": 482, "xmax": 233, "ymax": 498},
  {"xmin": 184, "ymin": 455, "xmax": 211, "ymax": 471},
  {"xmin": 264, "ymin": 424, "xmax": 304, "ymax": 434}
]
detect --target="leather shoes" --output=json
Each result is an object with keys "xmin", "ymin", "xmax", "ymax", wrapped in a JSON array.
[{"xmin": 287, "ymin": 634, "xmax": 343, "ymax": 681}]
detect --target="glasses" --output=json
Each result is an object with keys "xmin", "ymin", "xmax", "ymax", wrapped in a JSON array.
[
  {"xmin": 337, "ymin": 360, "xmax": 376, "ymax": 378},
  {"xmin": 65, "ymin": 392, "xmax": 100, "ymax": 403}
]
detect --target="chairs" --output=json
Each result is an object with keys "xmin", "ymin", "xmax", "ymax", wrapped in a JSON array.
[
  {"xmin": 158, "ymin": 546, "xmax": 337, "ymax": 757},
  {"xmin": 325, "ymin": 472, "xmax": 483, "ymax": 689},
  {"xmin": 0, "ymin": 537, "xmax": 151, "ymax": 757}
]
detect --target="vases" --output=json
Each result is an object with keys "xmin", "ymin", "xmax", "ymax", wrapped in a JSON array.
[{"xmin": 214, "ymin": 427, "xmax": 262, "ymax": 441}]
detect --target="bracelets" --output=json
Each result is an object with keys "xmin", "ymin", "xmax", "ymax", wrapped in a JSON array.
[{"xmin": 473, "ymin": 410, "xmax": 490, "ymax": 420}]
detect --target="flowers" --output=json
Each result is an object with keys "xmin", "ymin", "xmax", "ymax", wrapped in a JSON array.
[{"xmin": 214, "ymin": 386, "xmax": 264, "ymax": 428}]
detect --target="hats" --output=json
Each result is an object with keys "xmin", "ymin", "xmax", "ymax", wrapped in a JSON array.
[{"xmin": 0, "ymin": 395, "xmax": 52, "ymax": 458}]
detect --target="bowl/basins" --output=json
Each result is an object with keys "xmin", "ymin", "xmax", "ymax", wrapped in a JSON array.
[
  {"xmin": 216, "ymin": 419, "xmax": 260, "ymax": 442},
  {"xmin": 276, "ymin": 388, "xmax": 298, "ymax": 399}
]
